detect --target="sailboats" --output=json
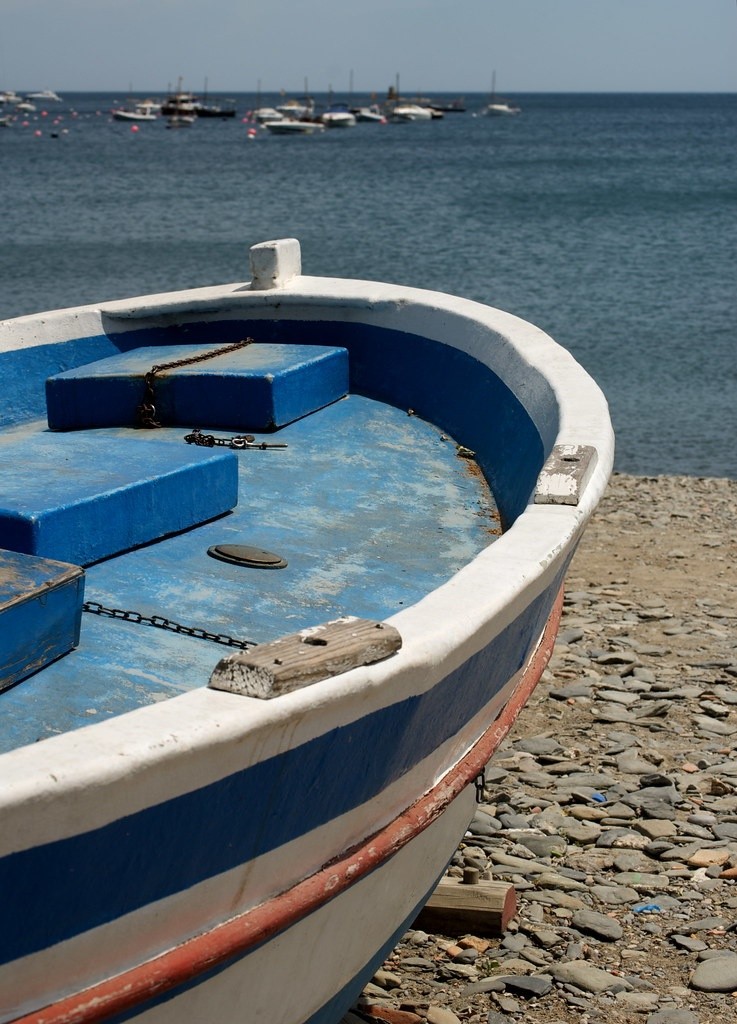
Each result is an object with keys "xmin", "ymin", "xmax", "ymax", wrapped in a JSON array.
[
  {"xmin": 296, "ymin": 78, "xmax": 314, "ymax": 99},
  {"xmin": 470, "ymin": 67, "xmax": 522, "ymax": 119}
]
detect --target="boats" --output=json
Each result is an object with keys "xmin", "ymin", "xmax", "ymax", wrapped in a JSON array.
[
  {"xmin": 354, "ymin": 110, "xmax": 386, "ymax": 122},
  {"xmin": 253, "ymin": 108, "xmax": 284, "ymax": 121},
  {"xmin": 423, "ymin": 107, "xmax": 444, "ymax": 119},
  {"xmin": 390, "ymin": 104, "xmax": 432, "ymax": 123},
  {"xmin": 17, "ymin": 103, "xmax": 37, "ymax": 112},
  {"xmin": 161, "ymin": 101, "xmax": 236, "ymax": 117},
  {"xmin": 134, "ymin": 99, "xmax": 161, "ymax": 110},
  {"xmin": 164, "ymin": 114, "xmax": 198, "ymax": 129},
  {"xmin": 275, "ymin": 102, "xmax": 316, "ymax": 118},
  {"xmin": 167, "ymin": 90, "xmax": 199, "ymax": 102},
  {"xmin": 0, "ymin": 118, "xmax": 11, "ymax": 127},
  {"xmin": 0, "ymin": 238, "xmax": 617, "ymax": 1024},
  {"xmin": 0, "ymin": 90, "xmax": 23, "ymax": 103},
  {"xmin": 114, "ymin": 107, "xmax": 157, "ymax": 120},
  {"xmin": 263, "ymin": 115, "xmax": 323, "ymax": 136},
  {"xmin": 25, "ymin": 89, "xmax": 63, "ymax": 103},
  {"xmin": 350, "ymin": 105, "xmax": 380, "ymax": 114},
  {"xmin": 428, "ymin": 102, "xmax": 467, "ymax": 113},
  {"xmin": 320, "ymin": 103, "xmax": 357, "ymax": 127}
]
